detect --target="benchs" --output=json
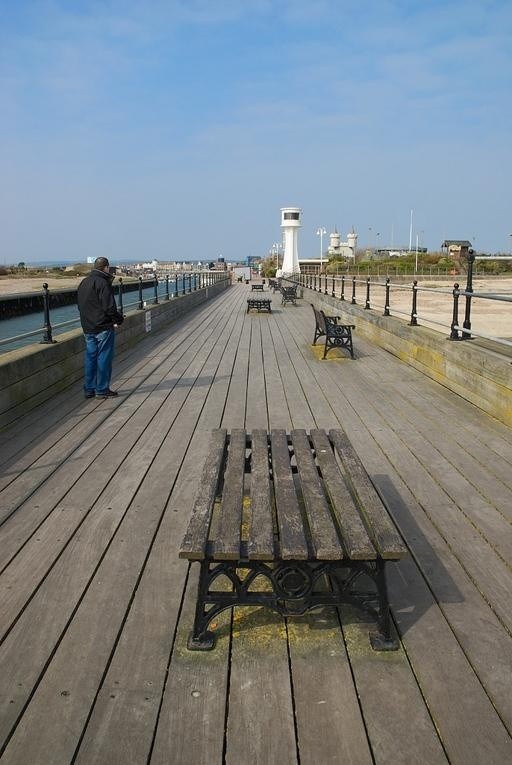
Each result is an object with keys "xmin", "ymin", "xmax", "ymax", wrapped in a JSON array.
[
  {"xmin": 179, "ymin": 428, "xmax": 408, "ymax": 652},
  {"xmin": 268, "ymin": 279, "xmax": 299, "ymax": 307},
  {"xmin": 247, "ymin": 297, "xmax": 272, "ymax": 314},
  {"xmin": 310, "ymin": 303, "xmax": 355, "ymax": 360},
  {"xmin": 251, "ymin": 284, "xmax": 264, "ymax": 292}
]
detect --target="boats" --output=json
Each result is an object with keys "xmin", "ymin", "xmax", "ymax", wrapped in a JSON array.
[{"xmin": 158, "ymin": 275, "xmax": 193, "ymax": 283}]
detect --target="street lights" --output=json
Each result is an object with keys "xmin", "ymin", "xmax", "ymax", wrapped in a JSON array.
[
  {"xmin": 416, "ymin": 230, "xmax": 426, "ymax": 273},
  {"xmin": 272, "ymin": 239, "xmax": 282, "ymax": 273},
  {"xmin": 376, "ymin": 232, "xmax": 381, "ymax": 258},
  {"xmin": 316, "ymin": 225, "xmax": 327, "ymax": 276},
  {"xmin": 268, "ymin": 247, "xmax": 277, "ymax": 262}
]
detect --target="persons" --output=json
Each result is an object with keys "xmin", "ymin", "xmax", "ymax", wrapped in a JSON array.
[{"xmin": 76, "ymin": 256, "xmax": 126, "ymax": 400}]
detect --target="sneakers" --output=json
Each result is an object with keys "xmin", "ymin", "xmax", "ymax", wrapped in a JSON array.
[
  {"xmin": 85, "ymin": 391, "xmax": 95, "ymax": 398},
  {"xmin": 95, "ymin": 391, "xmax": 118, "ymax": 400}
]
